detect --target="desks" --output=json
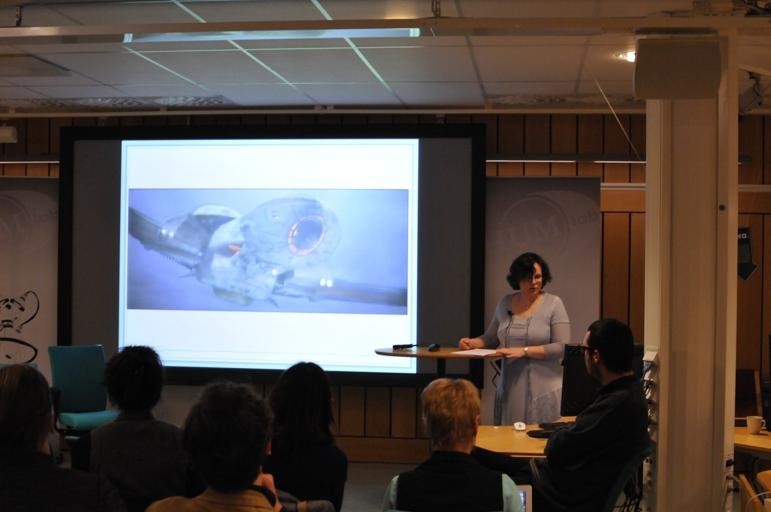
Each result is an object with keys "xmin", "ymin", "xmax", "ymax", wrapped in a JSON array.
[
  {"xmin": 374, "ymin": 346, "xmax": 507, "ymax": 378},
  {"xmin": 473, "ymin": 423, "xmax": 548, "ymax": 487},
  {"xmin": 732, "ymin": 426, "xmax": 770, "ymax": 474}
]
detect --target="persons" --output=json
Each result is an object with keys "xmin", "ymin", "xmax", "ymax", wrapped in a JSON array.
[
  {"xmin": 262, "ymin": 362, "xmax": 348, "ymax": 512},
  {"xmin": 146, "ymin": 382, "xmax": 288, "ymax": 512},
  {"xmin": 458, "ymin": 252, "xmax": 571, "ymax": 426},
  {"xmin": 382, "ymin": 378, "xmax": 524, "ymax": 512},
  {"xmin": 71, "ymin": 346, "xmax": 208, "ymax": 512},
  {"xmin": 544, "ymin": 319, "xmax": 647, "ymax": 512},
  {"xmin": 1, "ymin": 365, "xmax": 68, "ymax": 512}
]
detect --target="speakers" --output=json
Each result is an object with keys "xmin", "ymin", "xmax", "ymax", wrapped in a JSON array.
[{"xmin": 632, "ymin": 35, "xmax": 723, "ymax": 100}]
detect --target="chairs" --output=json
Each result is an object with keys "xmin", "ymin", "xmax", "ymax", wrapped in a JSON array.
[
  {"xmin": 48, "ymin": 343, "xmax": 121, "ymax": 439},
  {"xmin": 736, "ymin": 367, "xmax": 764, "ymax": 425}
]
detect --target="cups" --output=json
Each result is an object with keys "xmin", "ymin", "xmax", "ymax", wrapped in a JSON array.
[{"xmin": 747, "ymin": 416, "xmax": 766, "ymax": 434}]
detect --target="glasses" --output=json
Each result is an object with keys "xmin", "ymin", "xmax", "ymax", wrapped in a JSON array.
[{"xmin": 577, "ymin": 343, "xmax": 593, "ymax": 354}]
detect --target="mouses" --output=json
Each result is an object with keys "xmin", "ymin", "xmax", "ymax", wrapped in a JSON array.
[{"xmin": 428, "ymin": 343, "xmax": 442, "ymax": 351}]
